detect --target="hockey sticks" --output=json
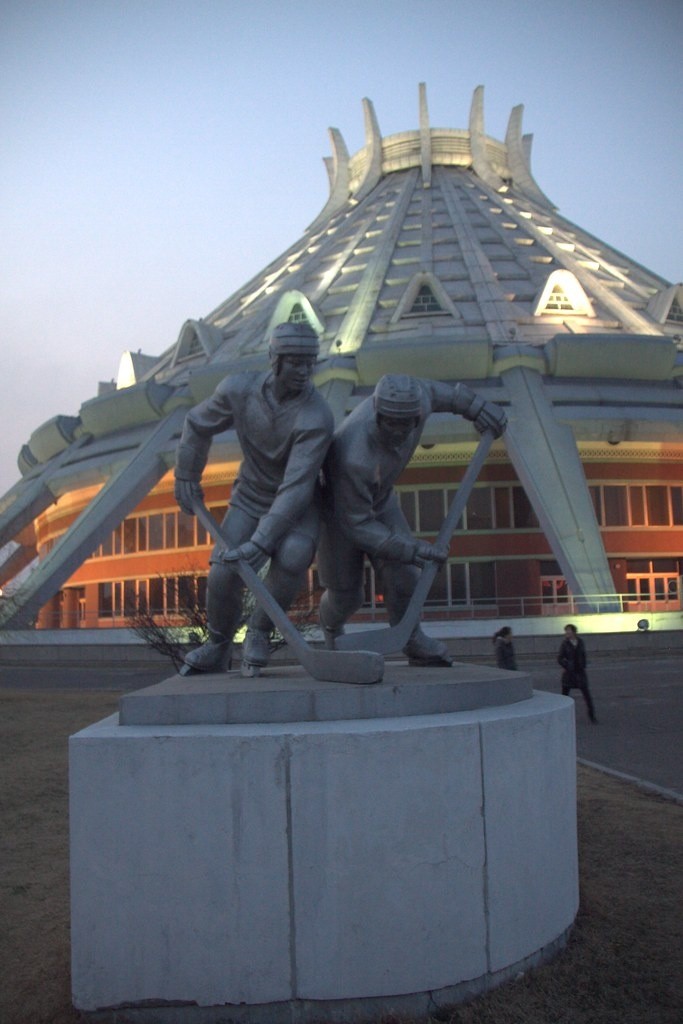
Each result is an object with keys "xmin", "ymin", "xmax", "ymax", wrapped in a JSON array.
[
  {"xmin": 335, "ymin": 430, "xmax": 493, "ymax": 657},
  {"xmin": 191, "ymin": 501, "xmax": 383, "ymax": 682}
]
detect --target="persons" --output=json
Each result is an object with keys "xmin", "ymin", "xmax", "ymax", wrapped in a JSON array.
[
  {"xmin": 181, "ymin": 321, "xmax": 337, "ymax": 679},
  {"xmin": 557, "ymin": 623, "xmax": 598, "ymax": 726},
  {"xmin": 491, "ymin": 624, "xmax": 522, "ymax": 671},
  {"xmin": 322, "ymin": 370, "xmax": 509, "ymax": 666}
]
